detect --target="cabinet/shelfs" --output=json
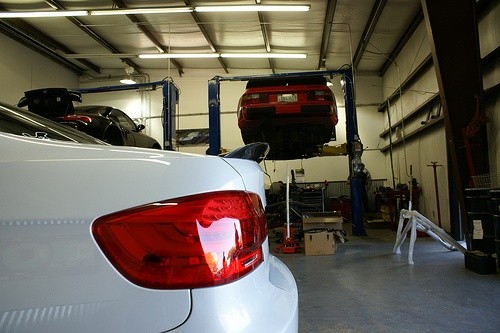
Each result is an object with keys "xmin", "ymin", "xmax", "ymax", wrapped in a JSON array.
[{"xmin": 465, "ymin": 188, "xmax": 500, "ymax": 268}]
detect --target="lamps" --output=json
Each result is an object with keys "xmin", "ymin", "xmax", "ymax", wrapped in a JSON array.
[
  {"xmin": 0, "ymin": 5, "xmax": 311, "ymax": 18},
  {"xmin": 120, "ymin": 66, "xmax": 136, "ymax": 84},
  {"xmin": 65, "ymin": 53, "xmax": 309, "ymax": 59}
]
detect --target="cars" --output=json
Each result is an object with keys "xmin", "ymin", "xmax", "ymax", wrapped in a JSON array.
[
  {"xmin": 0, "ymin": 101, "xmax": 298, "ymax": 333},
  {"xmin": 18, "ymin": 88, "xmax": 162, "ymax": 150},
  {"xmin": 237, "ymin": 76, "xmax": 338, "ymax": 145}
]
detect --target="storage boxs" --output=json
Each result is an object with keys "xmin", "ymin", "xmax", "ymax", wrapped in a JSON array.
[
  {"xmin": 304, "ymin": 232, "xmax": 335, "ymax": 256},
  {"xmin": 464, "ymin": 252, "xmax": 497, "ymax": 274}
]
